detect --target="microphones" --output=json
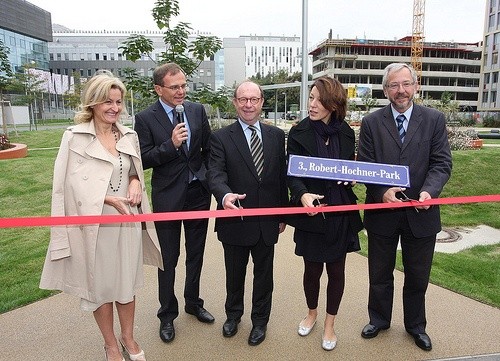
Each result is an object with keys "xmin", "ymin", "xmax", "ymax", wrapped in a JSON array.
[{"xmin": 176, "ymin": 105, "xmax": 186, "ymax": 144}]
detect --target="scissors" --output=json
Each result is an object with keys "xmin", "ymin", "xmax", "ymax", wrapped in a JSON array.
[
  {"xmin": 316, "ymin": 194, "xmax": 328, "ymax": 219},
  {"xmin": 235, "ymin": 194, "xmax": 245, "ymax": 221},
  {"xmin": 399, "ymin": 187, "xmax": 419, "ymax": 213},
  {"xmin": 126, "ymin": 198, "xmax": 135, "ymax": 216}
]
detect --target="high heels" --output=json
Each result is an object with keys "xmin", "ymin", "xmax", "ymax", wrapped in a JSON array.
[
  {"xmin": 103, "ymin": 344, "xmax": 125, "ymax": 361},
  {"xmin": 119, "ymin": 337, "xmax": 146, "ymax": 361}
]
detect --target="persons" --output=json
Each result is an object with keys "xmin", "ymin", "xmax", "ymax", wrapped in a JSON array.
[
  {"xmin": 208, "ymin": 81, "xmax": 290, "ymax": 345},
  {"xmin": 356, "ymin": 63, "xmax": 452, "ymax": 351},
  {"xmin": 36, "ymin": 76, "xmax": 165, "ymax": 361},
  {"xmin": 285, "ymin": 76, "xmax": 365, "ymax": 351},
  {"xmin": 134, "ymin": 63, "xmax": 216, "ymax": 343}
]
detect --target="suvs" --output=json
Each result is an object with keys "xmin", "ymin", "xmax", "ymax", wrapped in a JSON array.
[{"xmin": 282, "ymin": 112, "xmax": 296, "ymax": 120}]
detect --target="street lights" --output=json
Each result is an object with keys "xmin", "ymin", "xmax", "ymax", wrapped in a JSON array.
[
  {"xmin": 282, "ymin": 93, "xmax": 286, "ymax": 128},
  {"xmin": 27, "ymin": 60, "xmax": 35, "ymax": 126}
]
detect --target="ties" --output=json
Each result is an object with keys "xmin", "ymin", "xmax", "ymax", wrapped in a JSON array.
[
  {"xmin": 248, "ymin": 126, "xmax": 264, "ymax": 182},
  {"xmin": 172, "ymin": 109, "xmax": 189, "ymax": 157},
  {"xmin": 396, "ymin": 114, "xmax": 406, "ymax": 144}
]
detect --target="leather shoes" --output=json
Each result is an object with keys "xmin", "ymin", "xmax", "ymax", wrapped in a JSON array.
[
  {"xmin": 248, "ymin": 325, "xmax": 267, "ymax": 346},
  {"xmin": 160, "ymin": 319, "xmax": 176, "ymax": 344},
  {"xmin": 185, "ymin": 305, "xmax": 215, "ymax": 323},
  {"xmin": 223, "ymin": 317, "xmax": 241, "ymax": 338},
  {"xmin": 361, "ymin": 323, "xmax": 381, "ymax": 339},
  {"xmin": 406, "ymin": 329, "xmax": 432, "ymax": 351}
]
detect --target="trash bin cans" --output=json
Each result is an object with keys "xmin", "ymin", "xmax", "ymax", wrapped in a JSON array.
[{"xmin": 300, "ymin": 110, "xmax": 308, "ymax": 122}]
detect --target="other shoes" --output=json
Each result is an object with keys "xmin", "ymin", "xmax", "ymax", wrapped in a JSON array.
[
  {"xmin": 322, "ymin": 324, "xmax": 337, "ymax": 350},
  {"xmin": 298, "ymin": 314, "xmax": 318, "ymax": 336}
]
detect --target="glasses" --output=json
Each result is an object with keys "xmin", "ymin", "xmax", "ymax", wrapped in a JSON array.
[
  {"xmin": 389, "ymin": 81, "xmax": 415, "ymax": 89},
  {"xmin": 162, "ymin": 82, "xmax": 189, "ymax": 91},
  {"xmin": 238, "ymin": 96, "xmax": 262, "ymax": 105}
]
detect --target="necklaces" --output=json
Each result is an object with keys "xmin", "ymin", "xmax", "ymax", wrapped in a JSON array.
[{"xmin": 94, "ymin": 129, "xmax": 123, "ymax": 192}]
